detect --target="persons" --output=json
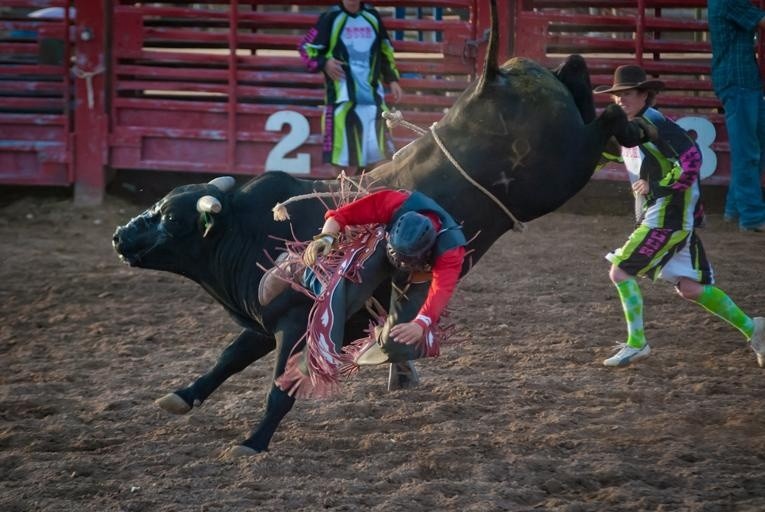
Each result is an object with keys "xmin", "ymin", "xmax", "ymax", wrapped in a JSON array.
[
  {"xmin": 257, "ymin": 189, "xmax": 468, "ymax": 363},
  {"xmin": 591, "ymin": 62, "xmax": 765, "ymax": 369},
  {"xmin": 708, "ymin": 0, "xmax": 765, "ymax": 234},
  {"xmin": 297, "ymin": 0, "xmax": 404, "ymax": 182}
]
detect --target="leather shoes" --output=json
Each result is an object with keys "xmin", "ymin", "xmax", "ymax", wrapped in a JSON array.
[{"xmin": 258, "ymin": 249, "xmax": 293, "ymax": 307}]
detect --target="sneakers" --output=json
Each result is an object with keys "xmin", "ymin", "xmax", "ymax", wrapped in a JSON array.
[
  {"xmin": 724, "ymin": 216, "xmax": 765, "ymax": 233},
  {"xmin": 751, "ymin": 316, "xmax": 765, "ymax": 368},
  {"xmin": 603, "ymin": 343, "xmax": 651, "ymax": 367}
]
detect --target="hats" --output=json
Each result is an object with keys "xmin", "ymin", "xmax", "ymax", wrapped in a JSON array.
[{"xmin": 594, "ymin": 64, "xmax": 665, "ymax": 95}]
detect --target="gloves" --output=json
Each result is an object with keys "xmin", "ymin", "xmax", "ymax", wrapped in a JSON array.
[{"xmin": 304, "ymin": 235, "xmax": 339, "ymax": 266}]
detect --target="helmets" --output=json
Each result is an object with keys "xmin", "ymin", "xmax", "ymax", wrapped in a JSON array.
[{"xmin": 390, "ymin": 211, "xmax": 437, "ymax": 257}]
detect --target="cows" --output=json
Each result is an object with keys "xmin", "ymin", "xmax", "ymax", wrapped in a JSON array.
[{"xmin": 107, "ymin": 1, "xmax": 663, "ymax": 461}]
